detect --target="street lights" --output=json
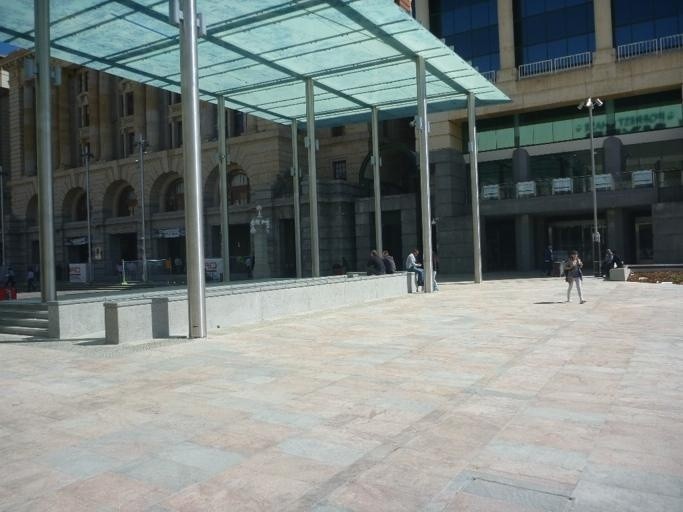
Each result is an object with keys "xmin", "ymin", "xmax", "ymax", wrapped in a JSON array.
[
  {"xmin": 577, "ymin": 95, "xmax": 606, "ymax": 276},
  {"xmin": 133, "ymin": 134, "xmax": 152, "ymax": 280},
  {"xmin": 81, "ymin": 145, "xmax": 95, "ymax": 283}
]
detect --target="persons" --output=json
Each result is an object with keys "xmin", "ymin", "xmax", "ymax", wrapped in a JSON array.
[
  {"xmin": 119, "ymin": 256, "xmax": 184, "ymax": 280},
  {"xmin": 368, "ymin": 247, "xmax": 439, "ymax": 291},
  {"xmin": 601, "ymin": 249, "xmax": 624, "ymax": 281},
  {"xmin": 543, "ymin": 243, "xmax": 554, "ymax": 275},
  {"xmin": 563, "ymin": 250, "xmax": 586, "ymax": 304},
  {"xmin": 27, "ymin": 269, "xmax": 36, "ymax": 292},
  {"xmin": 5, "ymin": 269, "xmax": 16, "ymax": 288},
  {"xmin": 245, "ymin": 257, "xmax": 254, "ymax": 279}
]
